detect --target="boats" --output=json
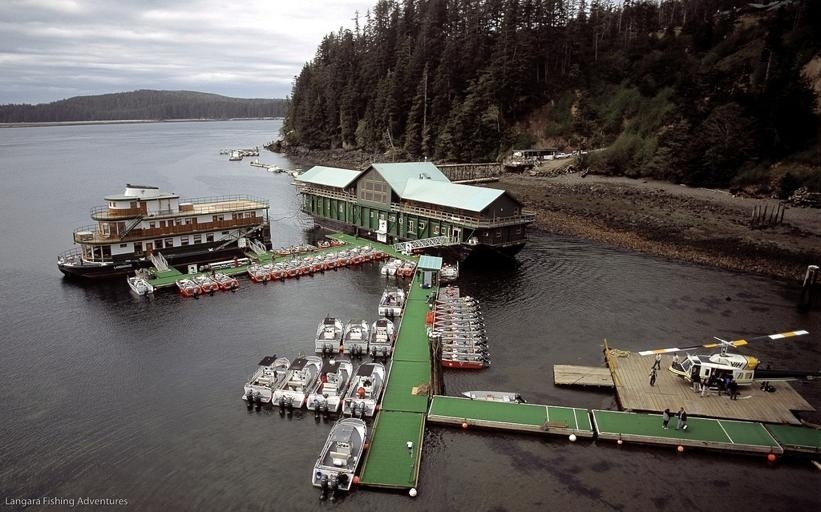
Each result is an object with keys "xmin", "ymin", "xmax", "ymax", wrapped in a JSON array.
[
  {"xmin": 126, "ymin": 274, "xmax": 157, "ymax": 297},
  {"xmin": 220, "ymin": 145, "xmax": 303, "ymax": 179},
  {"xmin": 435, "ymin": 259, "xmax": 492, "ymax": 370},
  {"xmin": 462, "ymin": 390, "xmax": 527, "ymax": 404},
  {"xmin": 57, "ymin": 183, "xmax": 273, "ymax": 279},
  {"xmin": 310, "ymin": 417, "xmax": 368, "ymax": 503}
]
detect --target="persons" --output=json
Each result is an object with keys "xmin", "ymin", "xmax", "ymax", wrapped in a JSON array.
[
  {"xmin": 650, "ymin": 354, "xmax": 661, "ymax": 370},
  {"xmin": 647, "ymin": 367, "xmax": 657, "ymax": 386},
  {"xmin": 661, "ymin": 409, "xmax": 672, "ymax": 430},
  {"xmin": 671, "ymin": 352, "xmax": 678, "ymax": 365},
  {"xmin": 760, "ymin": 364, "xmax": 771, "ymax": 387},
  {"xmin": 675, "ymin": 406, "xmax": 687, "ymax": 431},
  {"xmin": 691, "ymin": 366, "xmax": 738, "ymax": 402}
]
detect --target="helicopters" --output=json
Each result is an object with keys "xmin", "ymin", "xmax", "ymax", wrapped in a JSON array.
[{"xmin": 639, "ymin": 327, "xmax": 821, "ymax": 395}]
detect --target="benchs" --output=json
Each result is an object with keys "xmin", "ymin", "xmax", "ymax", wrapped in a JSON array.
[
  {"xmin": 330, "ymin": 451, "xmax": 351, "ymax": 466},
  {"xmin": 258, "ymin": 377, "xmax": 372, "ymax": 398}
]
